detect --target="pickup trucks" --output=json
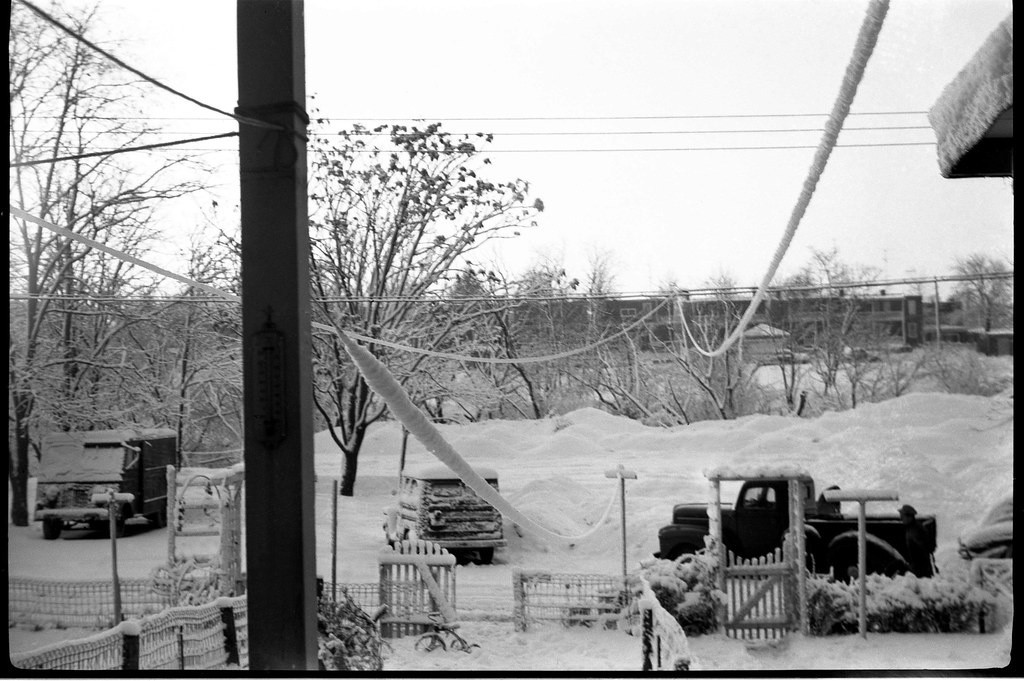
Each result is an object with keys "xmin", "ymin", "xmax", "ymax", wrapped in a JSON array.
[{"xmin": 652, "ymin": 470, "xmax": 937, "ymax": 583}]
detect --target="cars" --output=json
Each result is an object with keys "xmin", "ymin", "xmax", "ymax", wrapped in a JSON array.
[
  {"xmin": 382, "ymin": 469, "xmax": 508, "ymax": 566},
  {"xmin": 956, "ymin": 490, "xmax": 1014, "ymax": 571}
]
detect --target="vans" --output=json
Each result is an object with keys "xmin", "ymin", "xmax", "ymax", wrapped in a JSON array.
[{"xmin": 32, "ymin": 426, "xmax": 178, "ymax": 540}]
